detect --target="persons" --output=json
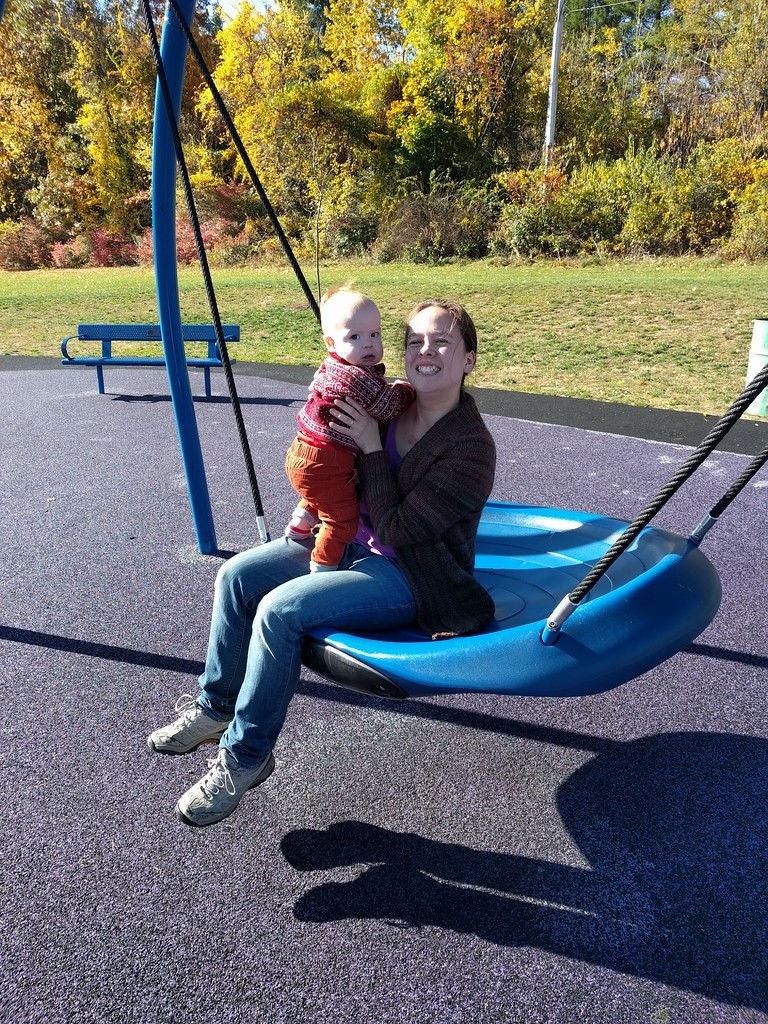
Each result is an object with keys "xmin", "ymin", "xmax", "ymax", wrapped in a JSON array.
[
  {"xmin": 285, "ymin": 288, "xmax": 415, "ymax": 573},
  {"xmin": 146, "ymin": 299, "xmax": 497, "ymax": 827}
]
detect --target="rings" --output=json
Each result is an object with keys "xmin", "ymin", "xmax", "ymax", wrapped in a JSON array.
[{"xmin": 349, "ymin": 419, "xmax": 353, "ymax": 426}]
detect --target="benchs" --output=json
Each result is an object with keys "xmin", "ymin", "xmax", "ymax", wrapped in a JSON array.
[{"xmin": 61, "ymin": 324, "xmax": 240, "ymax": 395}]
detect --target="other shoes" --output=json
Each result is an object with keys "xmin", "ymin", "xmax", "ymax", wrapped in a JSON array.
[{"xmin": 285, "ymin": 524, "xmax": 314, "ymax": 541}]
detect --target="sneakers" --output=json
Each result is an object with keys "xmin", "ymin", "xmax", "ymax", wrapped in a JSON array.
[
  {"xmin": 147, "ymin": 694, "xmax": 233, "ymax": 756},
  {"xmin": 175, "ymin": 749, "xmax": 275, "ymax": 827}
]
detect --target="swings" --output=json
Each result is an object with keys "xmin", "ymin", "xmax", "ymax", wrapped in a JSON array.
[{"xmin": 141, "ymin": 0, "xmax": 767, "ymax": 700}]
